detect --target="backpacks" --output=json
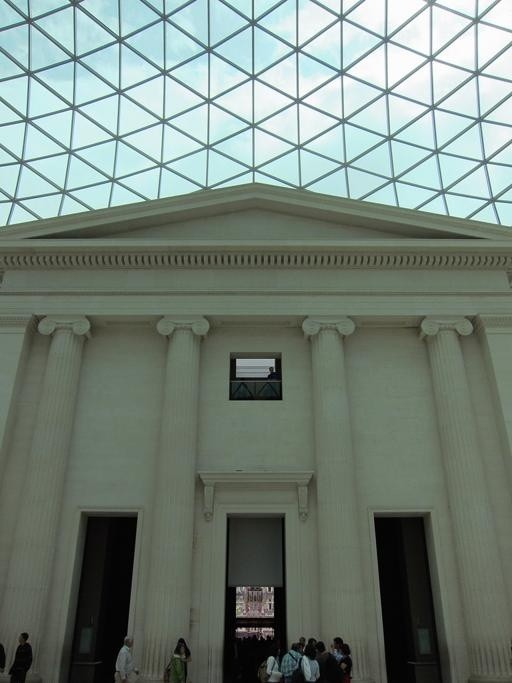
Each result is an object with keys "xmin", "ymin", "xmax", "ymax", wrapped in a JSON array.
[{"xmin": 258, "ymin": 659, "xmax": 280, "ymax": 683}]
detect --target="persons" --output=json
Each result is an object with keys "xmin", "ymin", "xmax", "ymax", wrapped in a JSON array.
[
  {"xmin": 7, "ymin": 632, "xmax": 34, "ymax": 683},
  {"xmin": 0, "ymin": 641, "xmax": 6, "ymax": 673},
  {"xmin": 266, "ymin": 364, "xmax": 277, "ymax": 380},
  {"xmin": 167, "ymin": 636, "xmax": 193, "ymax": 682},
  {"xmin": 237, "ymin": 632, "xmax": 353, "ymax": 682},
  {"xmin": 113, "ymin": 634, "xmax": 141, "ymax": 683}
]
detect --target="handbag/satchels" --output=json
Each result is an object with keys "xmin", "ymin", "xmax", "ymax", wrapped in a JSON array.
[{"xmin": 292, "ymin": 668, "xmax": 304, "ymax": 683}]
